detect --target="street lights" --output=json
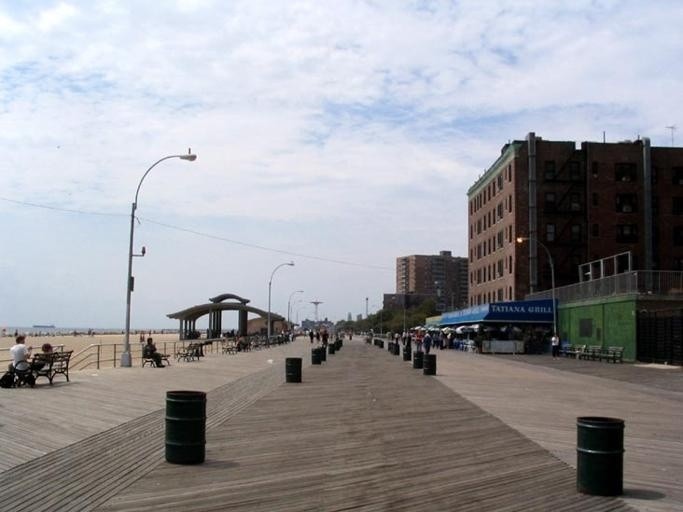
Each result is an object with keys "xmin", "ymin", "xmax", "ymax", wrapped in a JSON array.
[
  {"xmin": 288, "ymin": 288, "xmax": 307, "ymax": 333},
  {"xmin": 392, "ymin": 296, "xmax": 407, "ymax": 338},
  {"xmin": 516, "ymin": 236, "xmax": 561, "ymax": 354},
  {"xmin": 266, "ymin": 259, "xmax": 295, "ymax": 340},
  {"xmin": 369, "ymin": 303, "xmax": 382, "ymax": 337},
  {"xmin": 120, "ymin": 149, "xmax": 200, "ymax": 368}
]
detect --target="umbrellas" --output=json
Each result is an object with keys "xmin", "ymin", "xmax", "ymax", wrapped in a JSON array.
[{"xmin": 409, "ymin": 323, "xmax": 521, "ymax": 339}]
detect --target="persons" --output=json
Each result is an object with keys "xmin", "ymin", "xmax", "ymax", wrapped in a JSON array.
[
  {"xmin": 444, "ymin": 332, "xmax": 451, "ymax": 350},
  {"xmin": 438, "ymin": 330, "xmax": 443, "ymax": 350},
  {"xmin": 423, "ymin": 330, "xmax": 433, "ymax": 353},
  {"xmin": 400, "ymin": 329, "xmax": 405, "ymax": 345},
  {"xmin": 288, "ymin": 333, "xmax": 292, "ymax": 343},
  {"xmin": 550, "ymin": 332, "xmax": 560, "ymax": 361},
  {"xmin": 28, "ymin": 343, "xmax": 53, "ymax": 371},
  {"xmin": 393, "ymin": 331, "xmax": 400, "ymax": 345},
  {"xmin": 305, "ymin": 325, "xmax": 352, "ymax": 350},
  {"xmin": 144, "ymin": 337, "xmax": 165, "ymax": 368},
  {"xmin": 234, "ymin": 333, "xmax": 249, "ymax": 352},
  {"xmin": 406, "ymin": 331, "xmax": 411, "ymax": 346},
  {"xmin": 431, "ymin": 331, "xmax": 438, "ymax": 349},
  {"xmin": 6, "ymin": 334, "xmax": 31, "ymax": 371},
  {"xmin": 414, "ymin": 331, "xmax": 423, "ymax": 351}
]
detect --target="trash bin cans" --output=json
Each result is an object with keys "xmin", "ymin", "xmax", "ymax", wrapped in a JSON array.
[
  {"xmin": 374, "ymin": 339, "xmax": 384, "ymax": 348},
  {"xmin": 388, "ymin": 342, "xmax": 400, "ymax": 356},
  {"xmin": 403, "ymin": 346, "xmax": 411, "ymax": 361},
  {"xmin": 328, "ymin": 343, "xmax": 336, "ymax": 354},
  {"xmin": 285, "ymin": 357, "xmax": 302, "ymax": 383},
  {"xmin": 577, "ymin": 416, "xmax": 625, "ymax": 497},
  {"xmin": 413, "ymin": 351, "xmax": 424, "ymax": 369},
  {"xmin": 311, "ymin": 346, "xmax": 326, "ymax": 364},
  {"xmin": 165, "ymin": 390, "xmax": 207, "ymax": 464},
  {"xmin": 423, "ymin": 354, "xmax": 436, "ymax": 375},
  {"xmin": 335, "ymin": 339, "xmax": 343, "ymax": 350}
]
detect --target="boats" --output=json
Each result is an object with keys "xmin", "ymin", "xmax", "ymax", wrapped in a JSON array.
[{"xmin": 31, "ymin": 324, "xmax": 56, "ymax": 328}]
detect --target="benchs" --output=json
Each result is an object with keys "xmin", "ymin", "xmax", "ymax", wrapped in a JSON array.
[
  {"xmin": 6, "ymin": 350, "xmax": 74, "ymax": 389},
  {"xmin": 141, "ymin": 339, "xmax": 199, "ymax": 368},
  {"xmin": 557, "ymin": 343, "xmax": 624, "ymax": 364}
]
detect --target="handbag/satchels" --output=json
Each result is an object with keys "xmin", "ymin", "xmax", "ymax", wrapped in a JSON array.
[{"xmin": 0, "ymin": 372, "xmax": 14, "ymax": 388}]
